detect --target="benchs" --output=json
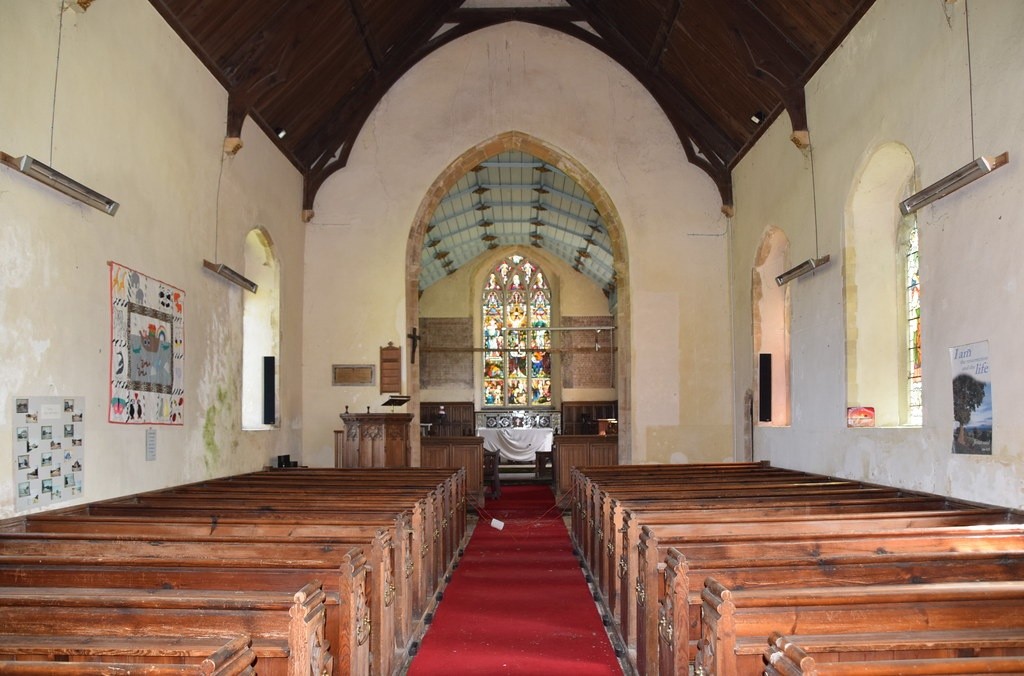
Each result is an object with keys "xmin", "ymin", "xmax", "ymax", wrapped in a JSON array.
[
  {"xmin": 0, "ymin": 465, "xmax": 466, "ymax": 676},
  {"xmin": 569, "ymin": 460, "xmax": 1024, "ymax": 676}
]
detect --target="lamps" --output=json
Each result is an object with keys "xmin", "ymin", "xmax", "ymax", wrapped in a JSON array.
[
  {"xmin": 898, "ymin": 0, "xmax": 992, "ymax": 216},
  {"xmin": 18, "ymin": 0, "xmax": 120, "ymax": 216},
  {"xmin": 776, "ymin": 143, "xmax": 819, "ymax": 287},
  {"xmin": 215, "ymin": 150, "xmax": 258, "ymax": 293}
]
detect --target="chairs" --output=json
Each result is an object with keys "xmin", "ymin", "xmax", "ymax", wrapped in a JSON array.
[{"xmin": 484, "ymin": 449, "xmax": 501, "ymax": 501}]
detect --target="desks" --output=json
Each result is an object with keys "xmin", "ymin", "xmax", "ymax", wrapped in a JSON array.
[{"xmin": 536, "ymin": 451, "xmax": 553, "ymax": 479}]
